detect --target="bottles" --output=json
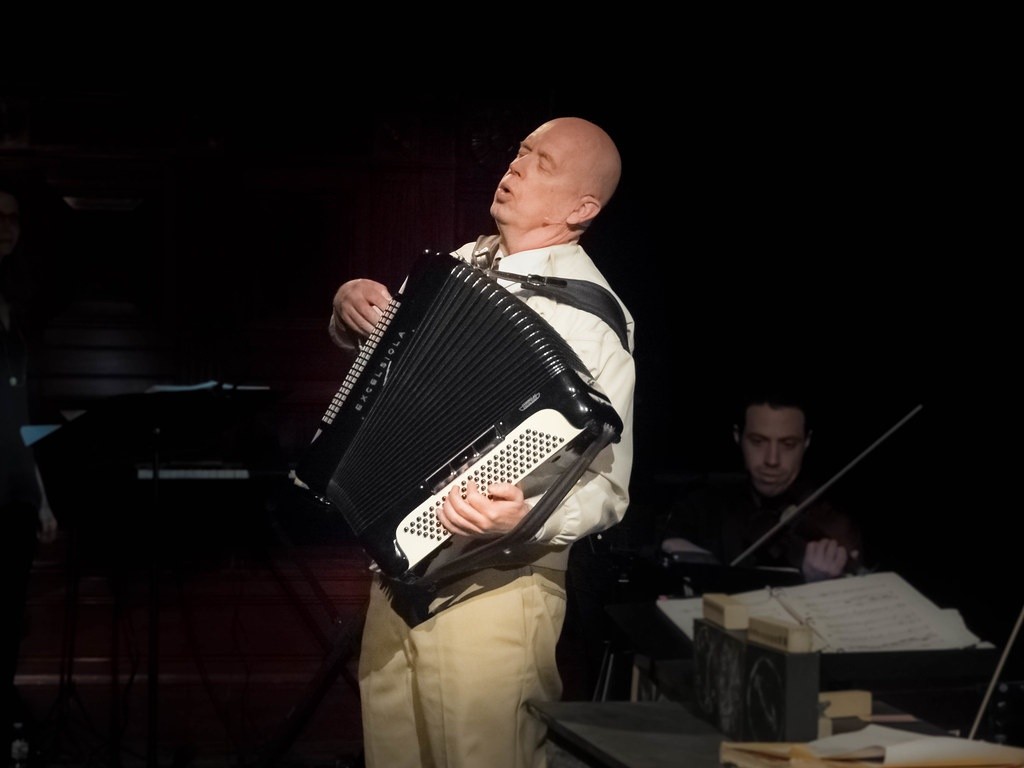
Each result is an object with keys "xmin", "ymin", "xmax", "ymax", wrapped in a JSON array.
[{"xmin": 10, "ymin": 722, "xmax": 28, "ymax": 768}]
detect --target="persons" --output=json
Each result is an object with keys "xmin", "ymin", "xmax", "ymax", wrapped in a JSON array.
[
  {"xmin": 637, "ymin": 377, "xmax": 862, "ymax": 702},
  {"xmin": 0, "ymin": 176, "xmax": 58, "ymax": 768},
  {"xmin": 327, "ymin": 117, "xmax": 638, "ymax": 768}
]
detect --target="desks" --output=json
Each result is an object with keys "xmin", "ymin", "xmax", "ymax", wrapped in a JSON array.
[{"xmin": 526, "ymin": 701, "xmax": 1024, "ymax": 768}]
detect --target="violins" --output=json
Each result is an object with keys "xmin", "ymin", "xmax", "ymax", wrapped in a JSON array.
[{"xmin": 737, "ymin": 496, "xmax": 866, "ymax": 577}]
